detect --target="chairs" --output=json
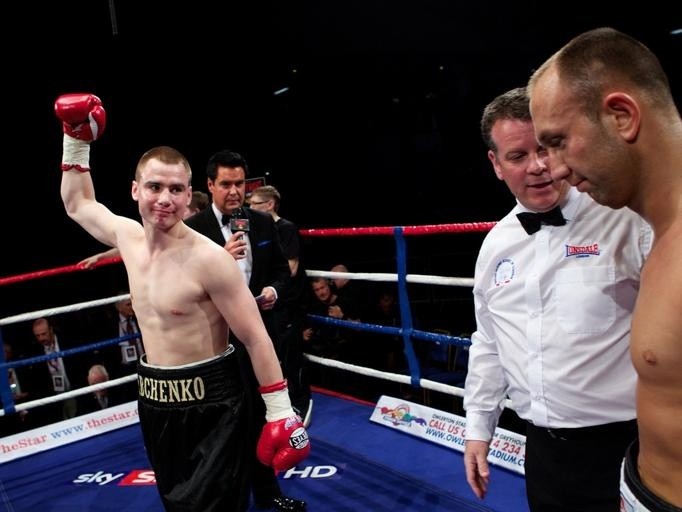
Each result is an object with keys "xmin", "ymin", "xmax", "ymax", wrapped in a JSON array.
[{"xmin": 397, "ymin": 330, "xmax": 472, "ymax": 406}]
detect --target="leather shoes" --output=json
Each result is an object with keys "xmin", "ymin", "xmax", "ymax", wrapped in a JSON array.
[{"xmin": 255, "ymin": 495, "xmax": 306, "ymax": 512}]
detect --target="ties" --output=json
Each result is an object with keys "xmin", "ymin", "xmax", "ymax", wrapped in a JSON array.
[{"xmin": 127, "ymin": 319, "xmax": 140, "ymax": 361}]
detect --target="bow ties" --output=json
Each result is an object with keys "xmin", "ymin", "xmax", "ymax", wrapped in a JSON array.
[
  {"xmin": 516, "ymin": 205, "xmax": 567, "ymax": 235},
  {"xmin": 222, "ymin": 214, "xmax": 249, "ymax": 226}
]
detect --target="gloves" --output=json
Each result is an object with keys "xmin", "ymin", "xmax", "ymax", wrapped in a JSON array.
[
  {"xmin": 54, "ymin": 94, "xmax": 105, "ymax": 171},
  {"xmin": 257, "ymin": 377, "xmax": 310, "ymax": 475}
]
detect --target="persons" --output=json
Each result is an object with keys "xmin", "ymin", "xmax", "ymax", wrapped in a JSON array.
[
  {"xmin": 520, "ymin": 23, "xmax": 681, "ymax": 510},
  {"xmin": 244, "ymin": 184, "xmax": 306, "ymax": 327},
  {"xmin": 178, "ymin": 147, "xmax": 301, "ymax": 438},
  {"xmin": 52, "ymin": 89, "xmax": 312, "ymax": 510},
  {"xmin": 23, "ymin": 317, "xmax": 93, "ymax": 420},
  {"xmin": 294, "ymin": 274, "xmax": 365, "ymax": 368},
  {"xmin": 326, "ymin": 263, "xmax": 394, "ymax": 370},
  {"xmin": 74, "ymin": 189, "xmax": 212, "ymax": 272},
  {"xmin": 459, "ymin": 85, "xmax": 659, "ymax": 512},
  {"xmin": 86, "ymin": 362, "xmax": 115, "ymax": 412},
  {"xmin": 106, "ymin": 290, "xmax": 148, "ymax": 388}
]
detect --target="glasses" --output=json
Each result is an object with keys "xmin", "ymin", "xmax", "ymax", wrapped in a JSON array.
[{"xmin": 248, "ymin": 199, "xmax": 271, "ymax": 207}]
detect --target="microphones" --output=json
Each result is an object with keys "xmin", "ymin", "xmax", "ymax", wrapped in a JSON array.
[{"xmin": 230, "ymin": 208, "xmax": 250, "ymax": 255}]
